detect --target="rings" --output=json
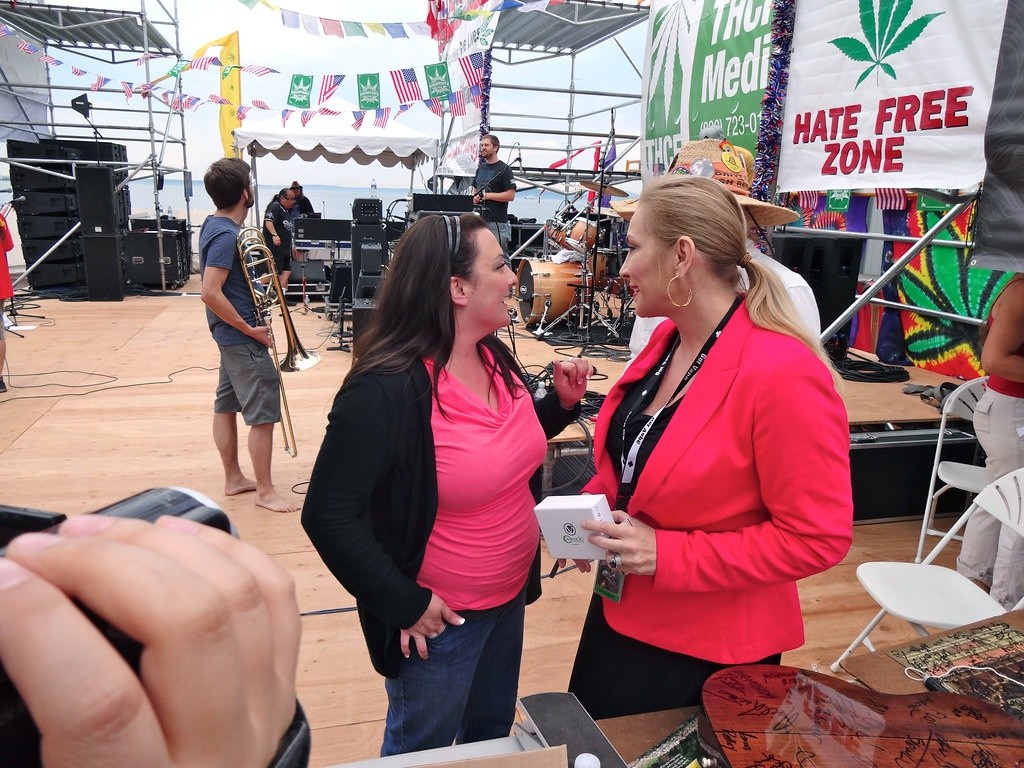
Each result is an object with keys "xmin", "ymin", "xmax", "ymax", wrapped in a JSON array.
[{"xmin": 609, "ymin": 554, "xmax": 622, "ymax": 569}]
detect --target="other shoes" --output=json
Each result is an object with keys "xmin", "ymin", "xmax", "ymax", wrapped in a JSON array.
[
  {"xmin": 0, "ymin": 376, "xmax": 7, "ymax": 393},
  {"xmin": 277, "ymin": 298, "xmax": 297, "ymax": 306}
]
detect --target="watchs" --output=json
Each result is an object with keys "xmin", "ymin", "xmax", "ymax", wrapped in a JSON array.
[{"xmin": 267, "ymin": 700, "xmax": 312, "ymax": 768}]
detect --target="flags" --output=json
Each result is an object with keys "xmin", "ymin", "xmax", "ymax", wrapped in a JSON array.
[
  {"xmin": 0, "ymin": 23, "xmax": 111, "ymax": 92},
  {"xmin": 236, "ymin": 100, "xmax": 271, "ymax": 120},
  {"xmin": 239, "ymin": 0, "xmax": 550, "ymax": 39},
  {"xmin": 121, "ymin": 81, "xmax": 133, "ymax": 105},
  {"xmin": 135, "ymin": 83, "xmax": 160, "ymax": 100},
  {"xmin": 281, "ymin": 51, "xmax": 484, "ymax": 130},
  {"xmin": 209, "ymin": 94, "xmax": 233, "ymax": 106},
  {"xmin": 167, "ymin": 57, "xmax": 280, "ymax": 79},
  {"xmin": 137, "ymin": 52, "xmax": 157, "ymax": 66},
  {"xmin": 162, "ymin": 90, "xmax": 200, "ymax": 111}
]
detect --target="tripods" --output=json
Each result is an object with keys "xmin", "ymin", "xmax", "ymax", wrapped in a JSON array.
[
  {"xmin": 537, "ymin": 129, "xmax": 636, "ymax": 358},
  {"xmin": 7, "ymin": 296, "xmax": 46, "ymax": 327}
]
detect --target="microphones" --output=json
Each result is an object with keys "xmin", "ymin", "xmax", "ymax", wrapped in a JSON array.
[
  {"xmin": 611, "ymin": 108, "xmax": 615, "ymax": 138},
  {"xmin": 518, "ymin": 143, "xmax": 523, "ymax": 173},
  {"xmin": 517, "ymin": 298, "xmax": 530, "ymax": 303},
  {"xmin": 607, "ymin": 274, "xmax": 614, "ymax": 300},
  {"xmin": 9, "ymin": 195, "xmax": 26, "ymax": 203},
  {"xmin": 567, "ymin": 200, "xmax": 579, "ymax": 215}
]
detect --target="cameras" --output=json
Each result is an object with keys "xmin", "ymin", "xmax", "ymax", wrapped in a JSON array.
[{"xmin": 0, "ymin": 485, "xmax": 240, "ymax": 768}]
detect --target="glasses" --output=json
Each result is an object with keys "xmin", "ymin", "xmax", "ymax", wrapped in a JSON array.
[
  {"xmin": 247, "ymin": 177, "xmax": 257, "ymax": 188},
  {"xmin": 282, "ymin": 194, "xmax": 296, "ymax": 204}
]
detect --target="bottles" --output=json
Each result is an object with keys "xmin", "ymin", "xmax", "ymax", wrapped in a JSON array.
[
  {"xmin": 159, "ymin": 205, "xmax": 163, "ymax": 216},
  {"xmin": 168, "ymin": 206, "xmax": 172, "ymax": 220},
  {"xmin": 370, "ymin": 178, "xmax": 377, "ymax": 198},
  {"xmin": 535, "ymin": 382, "xmax": 547, "ymax": 399}
]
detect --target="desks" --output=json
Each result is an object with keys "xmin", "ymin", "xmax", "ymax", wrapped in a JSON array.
[{"xmin": 838, "ymin": 609, "xmax": 1024, "ymax": 719}]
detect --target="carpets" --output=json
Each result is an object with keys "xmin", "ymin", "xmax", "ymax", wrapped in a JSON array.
[{"xmin": 526, "ymin": 316, "xmax": 634, "ymax": 346}]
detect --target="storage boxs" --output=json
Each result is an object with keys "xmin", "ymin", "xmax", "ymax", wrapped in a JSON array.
[{"xmin": 532, "ymin": 494, "xmax": 615, "ymax": 560}]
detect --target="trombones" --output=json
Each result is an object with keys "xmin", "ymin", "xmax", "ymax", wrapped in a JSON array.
[{"xmin": 235, "ymin": 227, "xmax": 322, "ymax": 458}]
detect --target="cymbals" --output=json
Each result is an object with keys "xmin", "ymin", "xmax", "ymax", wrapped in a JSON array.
[
  {"xmin": 580, "ymin": 181, "xmax": 629, "ymax": 197},
  {"xmin": 593, "ymin": 206, "xmax": 621, "ymax": 217}
]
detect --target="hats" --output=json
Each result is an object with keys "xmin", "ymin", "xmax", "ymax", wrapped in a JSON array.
[
  {"xmin": 289, "ymin": 181, "xmax": 303, "ymax": 189},
  {"xmin": 610, "ymin": 139, "xmax": 801, "ymax": 226},
  {"xmin": 697, "ymin": 125, "xmax": 726, "ymax": 140}
]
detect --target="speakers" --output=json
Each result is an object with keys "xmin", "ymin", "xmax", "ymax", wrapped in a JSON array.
[
  {"xmin": 7, "ymin": 136, "xmax": 189, "ymax": 301},
  {"xmin": 772, "ymin": 231, "xmax": 865, "ymax": 363}
]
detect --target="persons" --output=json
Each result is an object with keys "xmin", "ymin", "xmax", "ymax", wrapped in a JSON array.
[
  {"xmin": 300, "ymin": 213, "xmax": 596, "ymax": 757},
  {"xmin": 199, "ymin": 157, "xmax": 300, "ymax": 512},
  {"xmin": 568, "ymin": 139, "xmax": 852, "ymax": 719},
  {"xmin": 262, "ymin": 181, "xmax": 315, "ymax": 306},
  {"xmin": 0, "ymin": 210, "xmax": 14, "ymax": 392},
  {"xmin": 0, "ymin": 515, "xmax": 312, "ymax": 768},
  {"xmin": 472, "ymin": 135, "xmax": 517, "ymax": 300},
  {"xmin": 957, "ymin": 270, "xmax": 1024, "ymax": 611}
]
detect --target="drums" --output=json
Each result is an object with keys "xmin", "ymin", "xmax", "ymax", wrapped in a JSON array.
[
  {"xmin": 547, "ymin": 222, "xmax": 565, "ymax": 246},
  {"xmin": 516, "ymin": 258, "xmax": 592, "ymax": 327},
  {"xmin": 601, "ymin": 277, "xmax": 634, "ymax": 298},
  {"xmin": 565, "ymin": 216, "xmax": 604, "ymax": 253},
  {"xmin": 586, "ymin": 250, "xmax": 607, "ymax": 290},
  {"xmin": 554, "ymin": 220, "xmax": 572, "ymax": 250}
]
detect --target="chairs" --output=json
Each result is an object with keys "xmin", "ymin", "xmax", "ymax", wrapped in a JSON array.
[
  {"xmin": 915, "ymin": 375, "xmax": 988, "ymax": 565},
  {"xmin": 830, "ymin": 468, "xmax": 1024, "ymax": 674}
]
